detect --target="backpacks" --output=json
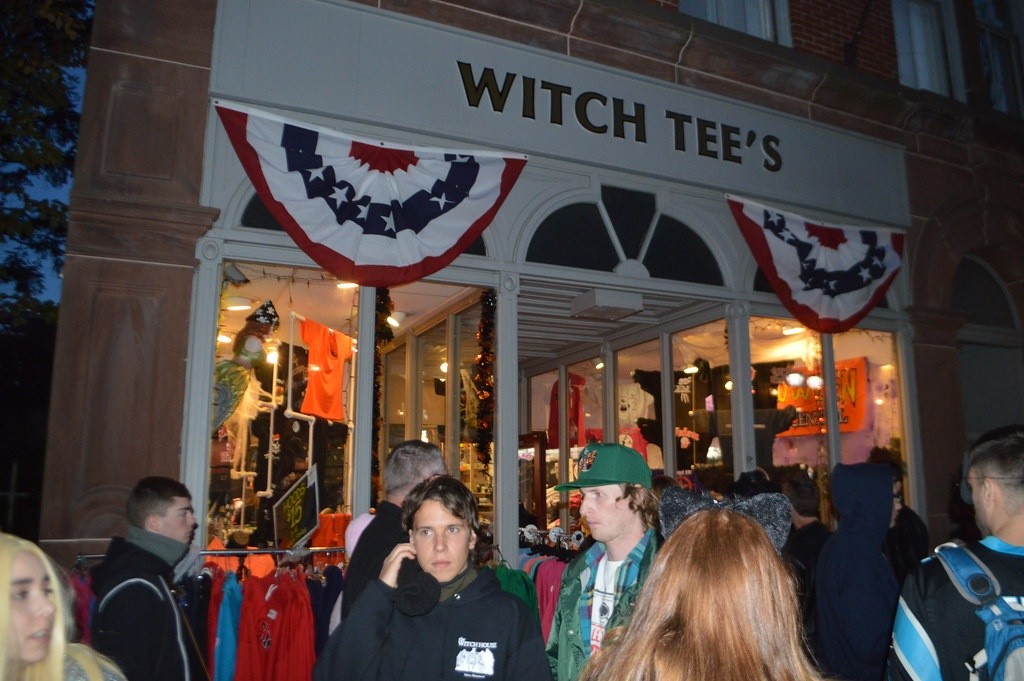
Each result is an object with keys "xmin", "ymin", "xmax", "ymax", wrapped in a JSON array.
[{"xmin": 935, "ymin": 540, "xmax": 1024, "ymax": 681}]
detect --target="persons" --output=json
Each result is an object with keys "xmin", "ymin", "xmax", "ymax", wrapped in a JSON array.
[
  {"xmin": 89, "ymin": 476, "xmax": 212, "ymax": 681},
  {"xmin": 311, "ymin": 474, "xmax": 555, "ymax": 681},
  {"xmin": 596, "ymin": 485, "xmax": 834, "ymax": 681},
  {"xmin": 544, "ymin": 442, "xmax": 681, "ymax": 681},
  {"xmin": 0, "ymin": 532, "xmax": 128, "ymax": 681},
  {"xmin": 885, "ymin": 424, "xmax": 1024, "ymax": 681},
  {"xmin": 340, "ymin": 440, "xmax": 447, "ymax": 621},
  {"xmin": 328, "ymin": 514, "xmax": 376, "ymax": 637},
  {"xmin": 781, "ymin": 446, "xmax": 929, "ymax": 681}
]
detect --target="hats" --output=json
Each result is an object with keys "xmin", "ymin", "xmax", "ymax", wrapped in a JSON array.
[{"xmin": 554, "ymin": 444, "xmax": 651, "ymax": 489}]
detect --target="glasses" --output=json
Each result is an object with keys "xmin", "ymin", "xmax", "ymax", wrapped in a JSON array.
[{"xmin": 892, "ymin": 493, "xmax": 903, "ymax": 504}]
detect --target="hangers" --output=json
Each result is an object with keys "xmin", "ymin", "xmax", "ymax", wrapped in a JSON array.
[
  {"xmin": 518, "ymin": 524, "xmax": 585, "ymax": 550},
  {"xmin": 75, "ymin": 546, "xmax": 346, "ymax": 582}
]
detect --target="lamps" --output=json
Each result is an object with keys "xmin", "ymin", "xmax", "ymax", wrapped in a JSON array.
[
  {"xmin": 217, "ymin": 331, "xmax": 233, "ymax": 343},
  {"xmin": 224, "ymin": 296, "xmax": 253, "ymax": 311},
  {"xmin": 387, "ymin": 311, "xmax": 407, "ymax": 327},
  {"xmin": 592, "ymin": 357, "xmax": 604, "ymax": 370},
  {"xmin": 680, "ymin": 364, "xmax": 699, "ymax": 374}
]
